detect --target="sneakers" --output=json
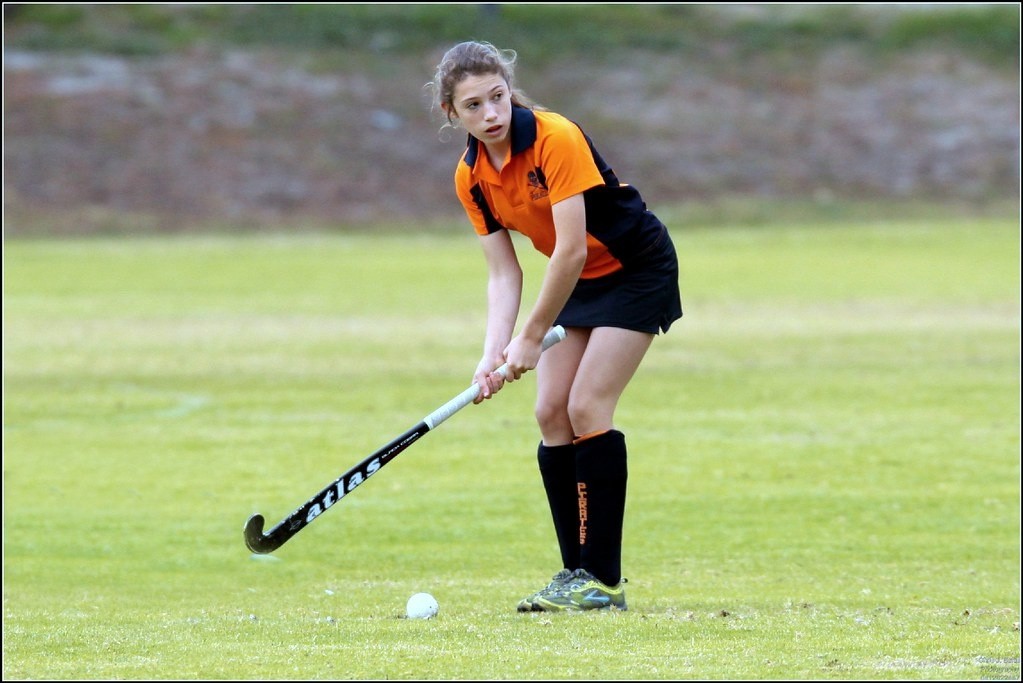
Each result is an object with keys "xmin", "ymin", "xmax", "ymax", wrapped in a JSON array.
[
  {"xmin": 531, "ymin": 570, "xmax": 628, "ymax": 611},
  {"xmin": 517, "ymin": 570, "xmax": 572, "ymax": 611}
]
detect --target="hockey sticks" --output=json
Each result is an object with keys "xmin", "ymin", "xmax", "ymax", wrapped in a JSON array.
[{"xmin": 242, "ymin": 323, "xmax": 570, "ymax": 556}]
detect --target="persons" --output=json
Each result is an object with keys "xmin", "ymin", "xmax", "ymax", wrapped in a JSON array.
[{"xmin": 434, "ymin": 38, "xmax": 686, "ymax": 613}]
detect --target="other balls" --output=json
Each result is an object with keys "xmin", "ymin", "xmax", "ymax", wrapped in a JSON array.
[{"xmin": 406, "ymin": 592, "xmax": 440, "ymax": 620}]
